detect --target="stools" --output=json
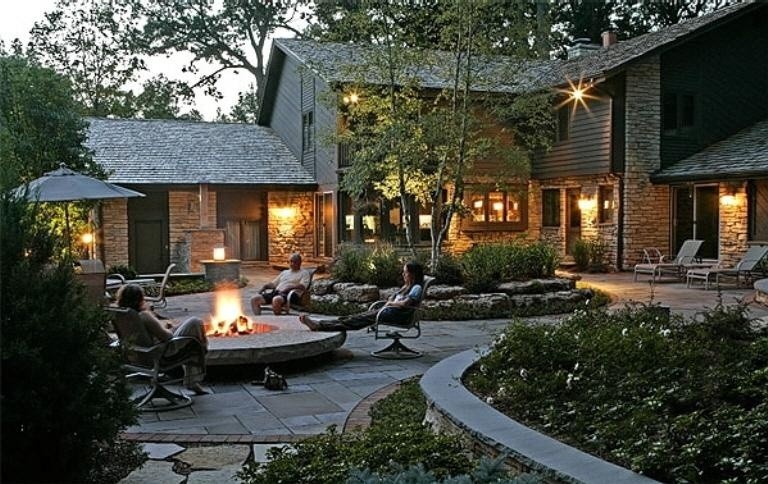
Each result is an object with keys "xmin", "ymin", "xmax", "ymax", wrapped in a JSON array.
[{"xmin": 753, "ymin": 277, "xmax": 768, "ymax": 307}]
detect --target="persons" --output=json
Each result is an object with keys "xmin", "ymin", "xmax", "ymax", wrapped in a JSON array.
[
  {"xmin": 250, "ymin": 253, "xmax": 310, "ymax": 316},
  {"xmin": 299, "ymin": 261, "xmax": 424, "ymax": 331},
  {"xmin": 115, "ymin": 282, "xmax": 212, "ymax": 396}
]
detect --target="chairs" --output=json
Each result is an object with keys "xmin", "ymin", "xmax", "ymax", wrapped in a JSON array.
[
  {"xmin": 367, "ymin": 274, "xmax": 435, "ymax": 361},
  {"xmin": 101, "ymin": 308, "xmax": 205, "ymax": 412},
  {"xmin": 632, "ymin": 238, "xmax": 705, "ymax": 283},
  {"xmin": 258, "ymin": 265, "xmax": 318, "ymax": 316},
  {"xmin": 685, "ymin": 244, "xmax": 768, "ymax": 290},
  {"xmin": 69, "ymin": 259, "xmax": 177, "ymax": 312}
]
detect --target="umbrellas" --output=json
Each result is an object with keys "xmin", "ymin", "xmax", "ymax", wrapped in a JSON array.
[{"xmin": 3, "ymin": 161, "xmax": 148, "ymax": 272}]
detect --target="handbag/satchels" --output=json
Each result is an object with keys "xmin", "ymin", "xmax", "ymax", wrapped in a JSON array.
[{"xmin": 265, "ymin": 367, "xmax": 287, "ymax": 391}]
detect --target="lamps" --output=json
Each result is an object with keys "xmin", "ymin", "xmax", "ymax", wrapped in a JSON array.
[
  {"xmin": 720, "ymin": 185, "xmax": 735, "ymax": 206},
  {"xmin": 578, "ymin": 191, "xmax": 587, "ymax": 209},
  {"xmin": 212, "ymin": 240, "xmax": 228, "ymax": 260}
]
